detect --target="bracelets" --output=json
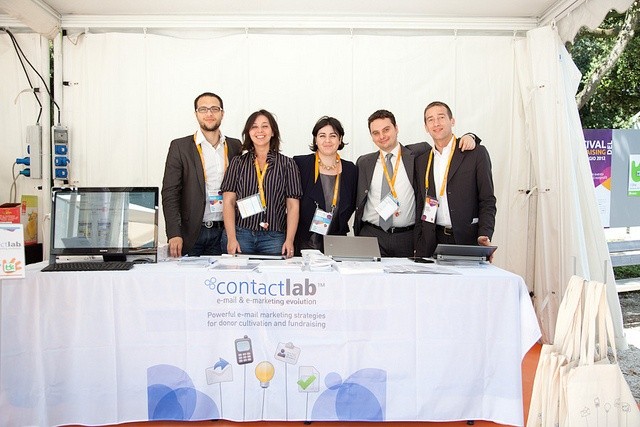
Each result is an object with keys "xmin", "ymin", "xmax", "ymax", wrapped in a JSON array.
[
  {"xmin": 477, "ymin": 236, "xmax": 490, "ymax": 246},
  {"xmin": 467, "ymin": 134, "xmax": 476, "ymax": 140}
]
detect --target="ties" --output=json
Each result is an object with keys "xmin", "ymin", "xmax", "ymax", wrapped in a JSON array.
[{"xmin": 378, "ymin": 153, "xmax": 394, "ymax": 233}]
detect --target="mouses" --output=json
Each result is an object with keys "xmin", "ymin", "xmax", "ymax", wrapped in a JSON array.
[{"xmin": 132, "ymin": 258, "xmax": 153, "ymax": 264}]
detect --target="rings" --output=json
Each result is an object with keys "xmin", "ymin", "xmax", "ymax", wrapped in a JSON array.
[{"xmin": 291, "ymin": 251, "xmax": 293, "ymax": 253}]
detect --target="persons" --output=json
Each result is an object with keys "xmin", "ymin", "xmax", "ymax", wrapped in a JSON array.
[
  {"xmin": 293, "ymin": 117, "xmax": 358, "ymax": 257},
  {"xmin": 413, "ymin": 102, "xmax": 497, "ymax": 261},
  {"xmin": 162, "ymin": 92, "xmax": 243, "ymax": 257},
  {"xmin": 353, "ymin": 110, "xmax": 481, "ymax": 259},
  {"xmin": 221, "ymin": 111, "xmax": 302, "ymax": 257}
]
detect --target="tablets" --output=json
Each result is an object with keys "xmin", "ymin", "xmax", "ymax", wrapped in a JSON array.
[{"xmin": 433, "ymin": 244, "xmax": 498, "ymax": 261}]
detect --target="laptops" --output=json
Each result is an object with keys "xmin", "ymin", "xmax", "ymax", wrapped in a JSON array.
[{"xmin": 324, "ymin": 235, "xmax": 382, "ymax": 262}]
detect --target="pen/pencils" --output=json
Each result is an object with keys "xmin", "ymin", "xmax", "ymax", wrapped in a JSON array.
[{"xmin": 211, "ymin": 259, "xmax": 219, "ymax": 264}]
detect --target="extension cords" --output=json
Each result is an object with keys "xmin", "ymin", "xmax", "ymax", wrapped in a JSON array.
[
  {"xmin": 52, "ymin": 125, "xmax": 70, "ymax": 181},
  {"xmin": 28, "ymin": 124, "xmax": 41, "ymax": 180}
]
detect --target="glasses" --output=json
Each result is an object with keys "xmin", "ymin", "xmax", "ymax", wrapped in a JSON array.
[{"xmin": 196, "ymin": 105, "xmax": 223, "ymax": 113}]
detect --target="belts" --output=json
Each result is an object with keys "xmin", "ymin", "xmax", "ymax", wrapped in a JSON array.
[
  {"xmin": 366, "ymin": 221, "xmax": 416, "ymax": 233},
  {"xmin": 436, "ymin": 224, "xmax": 453, "ymax": 235},
  {"xmin": 202, "ymin": 221, "xmax": 224, "ymax": 229}
]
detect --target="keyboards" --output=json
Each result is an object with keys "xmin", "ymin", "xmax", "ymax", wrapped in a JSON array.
[{"xmin": 41, "ymin": 262, "xmax": 134, "ymax": 270}]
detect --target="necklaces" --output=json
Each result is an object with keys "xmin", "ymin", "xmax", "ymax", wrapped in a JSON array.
[
  {"xmin": 212, "ymin": 130, "xmax": 221, "ymax": 147},
  {"xmin": 318, "ymin": 156, "xmax": 339, "ymax": 170}
]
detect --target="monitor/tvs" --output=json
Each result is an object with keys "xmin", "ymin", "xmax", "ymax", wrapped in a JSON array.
[{"xmin": 50, "ymin": 187, "xmax": 159, "ymax": 262}]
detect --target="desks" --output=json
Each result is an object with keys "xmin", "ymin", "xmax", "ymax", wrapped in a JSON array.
[{"xmin": 0, "ymin": 254, "xmax": 542, "ymax": 427}]
